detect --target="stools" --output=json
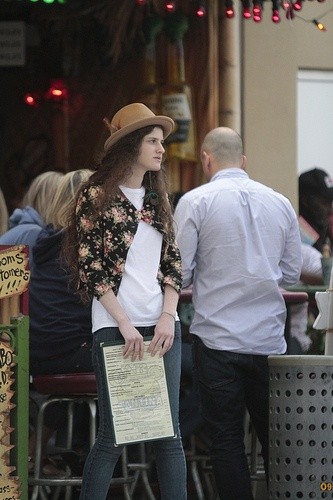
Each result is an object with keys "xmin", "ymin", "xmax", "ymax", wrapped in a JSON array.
[{"xmin": 32, "ymin": 372, "xmax": 257, "ymax": 500}]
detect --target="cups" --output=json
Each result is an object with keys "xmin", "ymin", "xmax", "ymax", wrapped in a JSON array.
[{"xmin": 321, "ymin": 257, "xmax": 333, "ymax": 287}]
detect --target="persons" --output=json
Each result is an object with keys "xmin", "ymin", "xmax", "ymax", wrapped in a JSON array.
[
  {"xmin": 28, "ymin": 168, "xmax": 121, "ymax": 478},
  {"xmin": 75, "ymin": 102, "xmax": 187, "ymax": 500},
  {"xmin": 174, "ymin": 126, "xmax": 303, "ymax": 500},
  {"xmin": 0, "ymin": 171, "xmax": 69, "ymax": 473},
  {"xmin": 298, "ymin": 168, "xmax": 333, "ymax": 253}
]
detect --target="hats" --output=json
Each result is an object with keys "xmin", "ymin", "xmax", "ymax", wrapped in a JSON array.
[
  {"xmin": 299, "ymin": 169, "xmax": 333, "ymax": 203},
  {"xmin": 103, "ymin": 102, "xmax": 176, "ymax": 152}
]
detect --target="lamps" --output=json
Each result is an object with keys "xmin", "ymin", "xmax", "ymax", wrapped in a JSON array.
[{"xmin": 166, "ymin": 0, "xmax": 325, "ymax": 23}]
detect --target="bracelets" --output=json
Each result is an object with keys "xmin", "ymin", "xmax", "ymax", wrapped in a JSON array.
[{"xmin": 162, "ymin": 312, "xmax": 177, "ymax": 320}]
